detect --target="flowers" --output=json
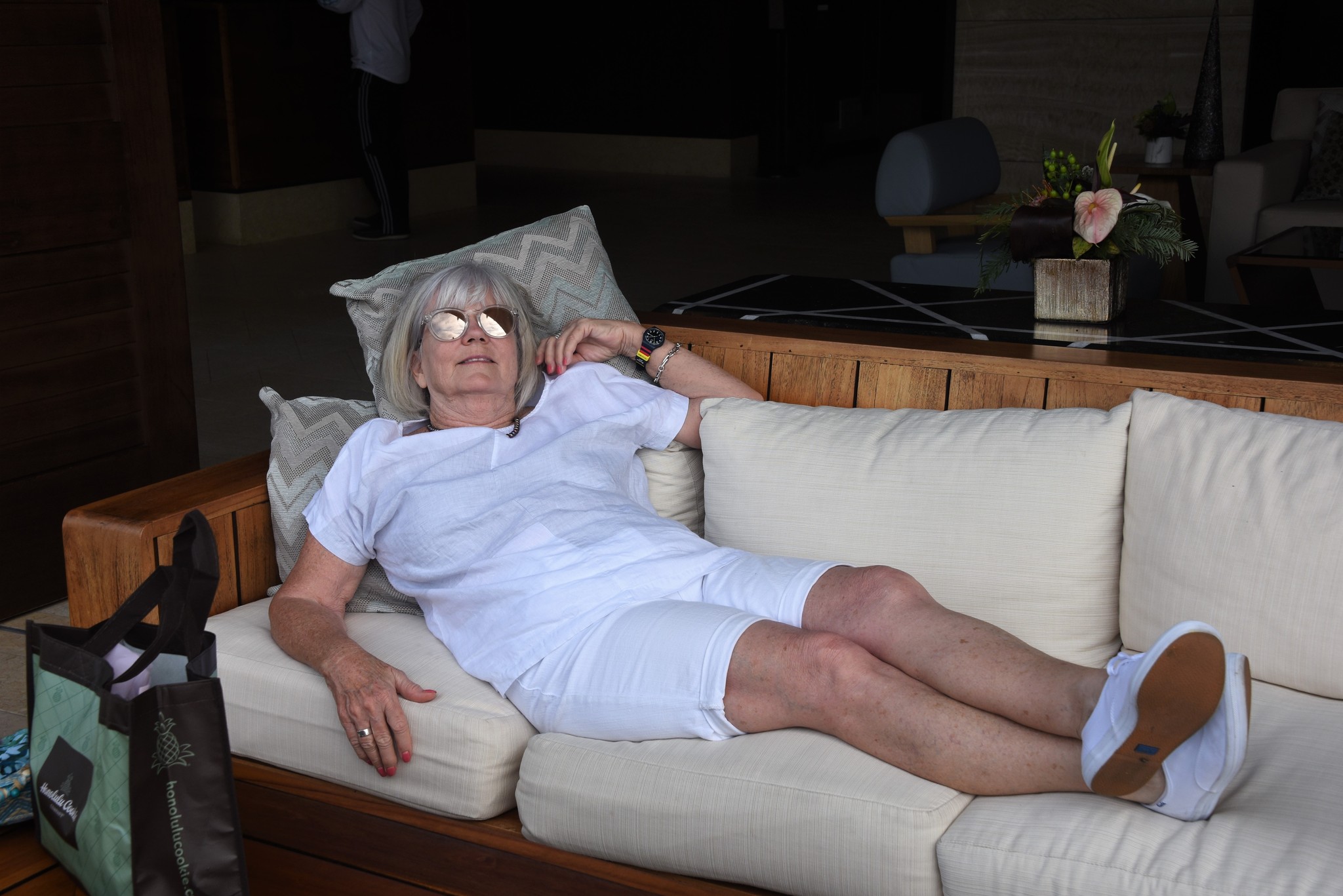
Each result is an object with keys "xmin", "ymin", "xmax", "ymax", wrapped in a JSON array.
[{"xmin": 959, "ymin": 118, "xmax": 1196, "ymax": 262}]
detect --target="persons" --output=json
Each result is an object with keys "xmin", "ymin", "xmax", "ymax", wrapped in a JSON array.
[
  {"xmin": 316, "ymin": 0, "xmax": 424, "ymax": 241},
  {"xmin": 267, "ymin": 263, "xmax": 1255, "ymax": 824}
]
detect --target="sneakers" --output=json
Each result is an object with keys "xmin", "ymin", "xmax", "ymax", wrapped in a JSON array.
[
  {"xmin": 1079, "ymin": 620, "xmax": 1224, "ymax": 795},
  {"xmin": 1146, "ymin": 653, "xmax": 1254, "ymax": 823}
]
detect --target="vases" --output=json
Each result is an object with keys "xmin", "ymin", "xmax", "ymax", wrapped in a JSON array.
[{"xmin": 1032, "ymin": 250, "xmax": 1130, "ymax": 322}]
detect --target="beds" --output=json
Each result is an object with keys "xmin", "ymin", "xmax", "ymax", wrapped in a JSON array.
[{"xmin": 64, "ymin": 395, "xmax": 1343, "ymax": 896}]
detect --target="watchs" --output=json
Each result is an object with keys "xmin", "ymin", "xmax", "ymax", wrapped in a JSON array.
[{"xmin": 634, "ymin": 326, "xmax": 666, "ymax": 371}]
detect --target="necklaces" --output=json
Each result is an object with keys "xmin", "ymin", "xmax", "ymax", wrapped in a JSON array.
[{"xmin": 426, "ymin": 414, "xmax": 521, "ymax": 441}]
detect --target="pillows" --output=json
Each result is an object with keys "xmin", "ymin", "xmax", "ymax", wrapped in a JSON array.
[
  {"xmin": 331, "ymin": 198, "xmax": 650, "ymax": 422},
  {"xmin": 1120, "ymin": 378, "xmax": 1343, "ymax": 704},
  {"xmin": 254, "ymin": 385, "xmax": 424, "ymax": 618},
  {"xmin": 695, "ymin": 387, "xmax": 1126, "ymax": 669}
]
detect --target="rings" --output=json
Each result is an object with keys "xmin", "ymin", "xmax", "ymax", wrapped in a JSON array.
[
  {"xmin": 357, "ymin": 727, "xmax": 373, "ymax": 738},
  {"xmin": 552, "ymin": 330, "xmax": 563, "ymax": 339}
]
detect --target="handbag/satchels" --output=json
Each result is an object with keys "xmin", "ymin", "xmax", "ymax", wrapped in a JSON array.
[{"xmin": 24, "ymin": 511, "xmax": 252, "ymax": 896}]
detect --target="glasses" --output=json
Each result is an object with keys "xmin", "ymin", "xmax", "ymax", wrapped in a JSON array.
[{"xmin": 416, "ymin": 305, "xmax": 518, "ymax": 350}]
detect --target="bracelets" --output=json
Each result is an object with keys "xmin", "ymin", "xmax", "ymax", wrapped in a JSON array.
[{"xmin": 653, "ymin": 342, "xmax": 683, "ymax": 383}]
